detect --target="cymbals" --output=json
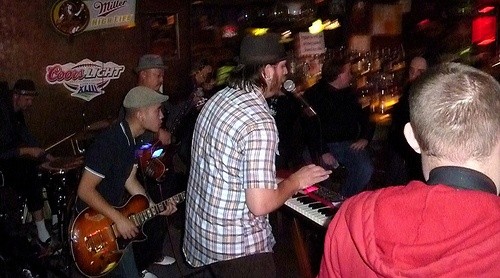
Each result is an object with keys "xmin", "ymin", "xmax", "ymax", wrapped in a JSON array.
[{"xmin": 87, "ymin": 119, "xmax": 119, "ymax": 132}]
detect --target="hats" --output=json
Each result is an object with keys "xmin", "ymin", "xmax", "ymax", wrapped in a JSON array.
[
  {"xmin": 134, "ymin": 54, "xmax": 169, "ymax": 73},
  {"xmin": 233, "ymin": 36, "xmax": 288, "ymax": 63},
  {"xmin": 12, "ymin": 79, "xmax": 39, "ymax": 97},
  {"xmin": 122, "ymin": 87, "xmax": 169, "ymax": 107}
]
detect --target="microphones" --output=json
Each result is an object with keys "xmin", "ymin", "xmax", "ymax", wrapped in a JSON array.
[{"xmin": 284, "ymin": 80, "xmax": 317, "ymax": 116}]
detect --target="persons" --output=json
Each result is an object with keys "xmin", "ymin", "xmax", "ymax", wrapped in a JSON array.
[
  {"xmin": 181, "ymin": 34, "xmax": 332, "ymax": 278},
  {"xmin": 0, "ymin": 54, "xmax": 376, "ymax": 278},
  {"xmin": 77, "ymin": 86, "xmax": 176, "ymax": 278},
  {"xmin": 315, "ymin": 62, "xmax": 500, "ymax": 278},
  {"xmin": 387, "ymin": 52, "xmax": 436, "ymax": 183}
]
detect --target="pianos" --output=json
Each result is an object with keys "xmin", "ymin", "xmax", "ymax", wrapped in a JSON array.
[{"xmin": 282, "ymin": 185, "xmax": 352, "ymax": 227}]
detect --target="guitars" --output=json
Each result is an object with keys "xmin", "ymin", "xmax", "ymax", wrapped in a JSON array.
[
  {"xmin": 138, "ymin": 89, "xmax": 203, "ymax": 187},
  {"xmin": 69, "ymin": 187, "xmax": 185, "ymax": 278}
]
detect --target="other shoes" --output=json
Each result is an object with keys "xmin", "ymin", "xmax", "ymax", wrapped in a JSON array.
[
  {"xmin": 138, "ymin": 270, "xmax": 157, "ymax": 278},
  {"xmin": 154, "ymin": 256, "xmax": 175, "ymax": 265}
]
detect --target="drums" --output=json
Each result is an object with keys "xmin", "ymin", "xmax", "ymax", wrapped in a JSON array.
[{"xmin": 39, "ymin": 156, "xmax": 84, "ymax": 203}]
